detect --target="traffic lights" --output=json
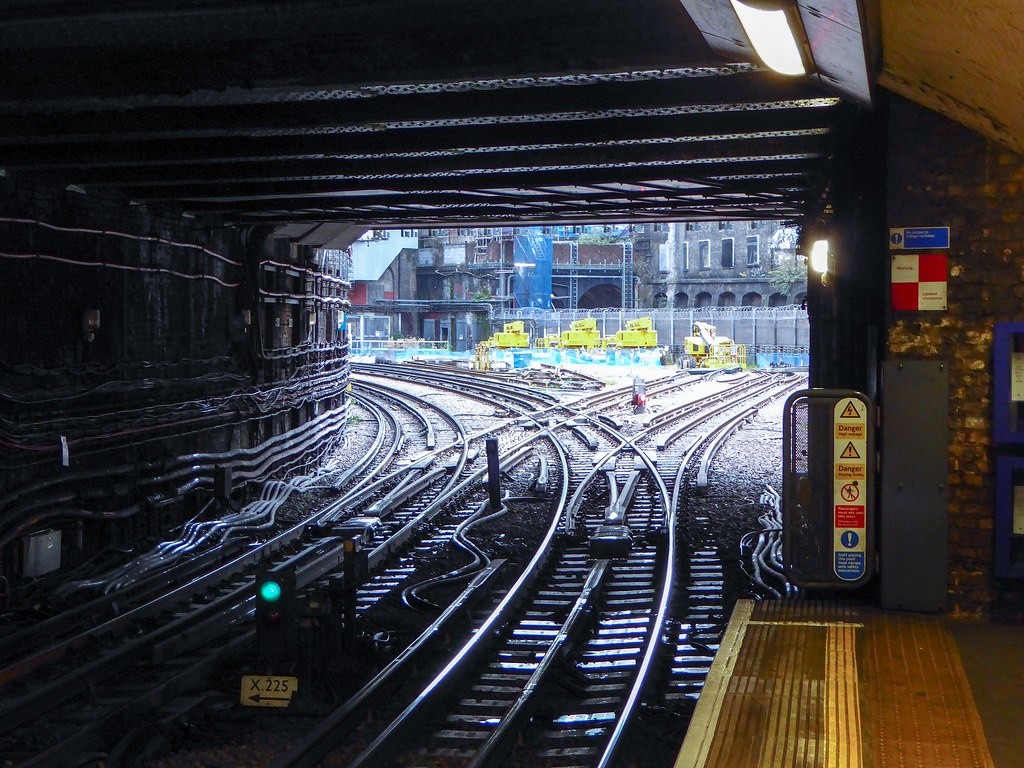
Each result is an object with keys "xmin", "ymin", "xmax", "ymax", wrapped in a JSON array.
[{"xmin": 255, "ymin": 571, "xmax": 296, "ymax": 615}]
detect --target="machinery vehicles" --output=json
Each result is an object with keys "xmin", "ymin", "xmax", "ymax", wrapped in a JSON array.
[{"xmin": 679, "ymin": 321, "xmax": 747, "ymax": 369}]
